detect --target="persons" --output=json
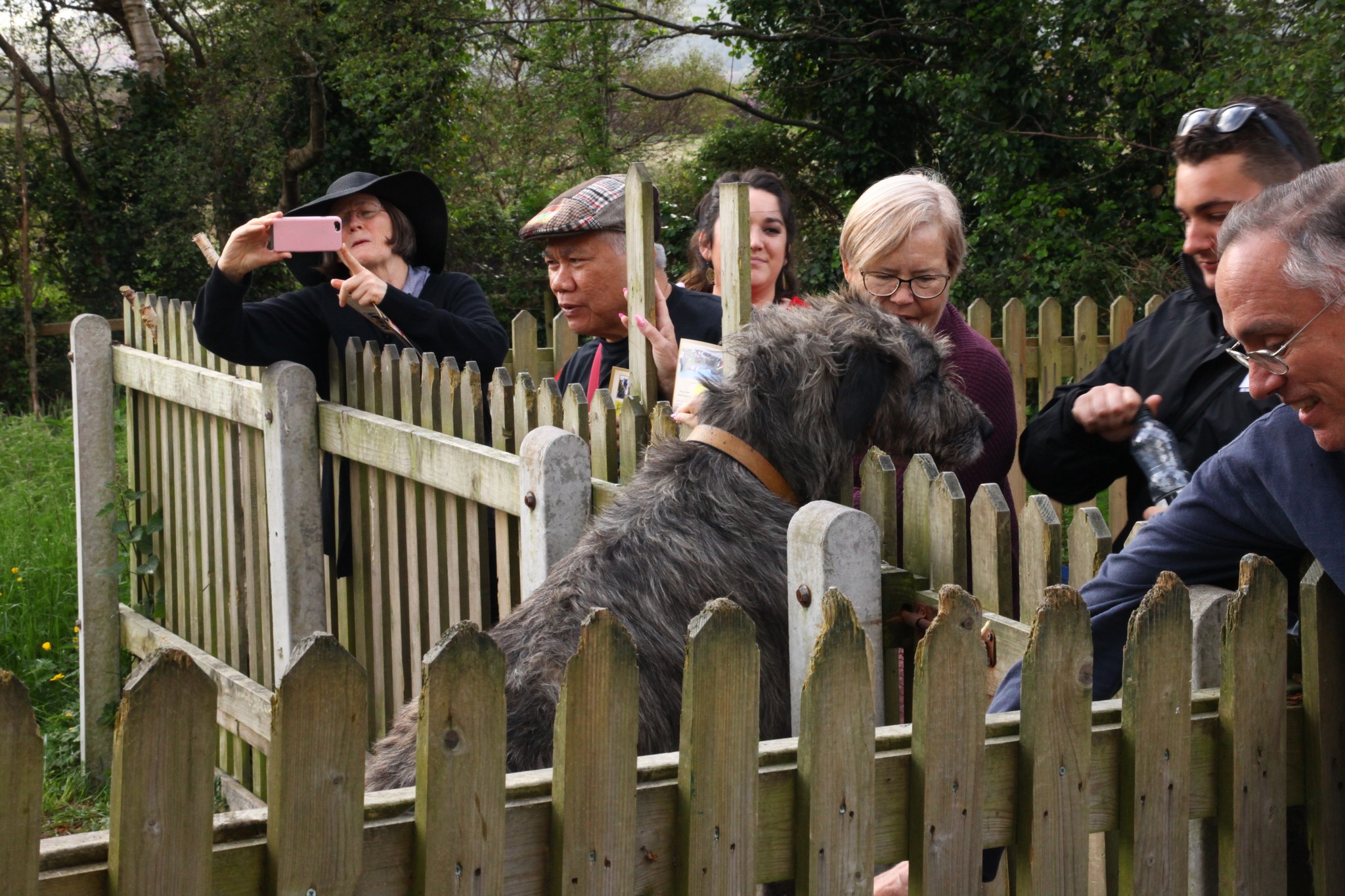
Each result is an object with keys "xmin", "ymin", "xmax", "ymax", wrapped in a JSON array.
[
  {"xmin": 616, "ymin": 165, "xmax": 1021, "ymax": 719},
  {"xmin": 674, "ymin": 170, "xmax": 814, "ymax": 314},
  {"xmin": 522, "ymin": 168, "xmax": 757, "ymax": 483},
  {"xmin": 862, "ymin": 141, "xmax": 1345, "ymax": 896},
  {"xmin": 1021, "ymin": 90, "xmax": 1317, "ymax": 625},
  {"xmin": 192, "ymin": 168, "xmax": 511, "ymax": 580}
]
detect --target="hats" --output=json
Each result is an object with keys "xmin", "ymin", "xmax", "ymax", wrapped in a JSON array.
[
  {"xmin": 517, "ymin": 172, "xmax": 661, "ymax": 243},
  {"xmin": 273, "ymin": 169, "xmax": 448, "ymax": 287}
]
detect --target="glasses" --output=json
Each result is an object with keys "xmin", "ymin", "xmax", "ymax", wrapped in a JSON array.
[
  {"xmin": 1226, "ymin": 292, "xmax": 1345, "ymax": 374},
  {"xmin": 860, "ymin": 264, "xmax": 951, "ymax": 301},
  {"xmin": 340, "ymin": 203, "xmax": 382, "ymax": 227},
  {"xmin": 1176, "ymin": 103, "xmax": 1298, "ymax": 162}
]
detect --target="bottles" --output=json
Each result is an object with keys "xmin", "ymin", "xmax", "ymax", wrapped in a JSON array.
[{"xmin": 1130, "ymin": 402, "xmax": 1193, "ymax": 505}]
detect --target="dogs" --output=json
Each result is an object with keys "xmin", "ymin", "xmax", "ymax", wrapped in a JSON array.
[{"xmin": 361, "ymin": 277, "xmax": 1007, "ymax": 797}]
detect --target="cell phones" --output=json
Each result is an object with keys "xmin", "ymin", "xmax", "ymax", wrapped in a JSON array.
[{"xmin": 272, "ymin": 217, "xmax": 344, "ymax": 252}]
